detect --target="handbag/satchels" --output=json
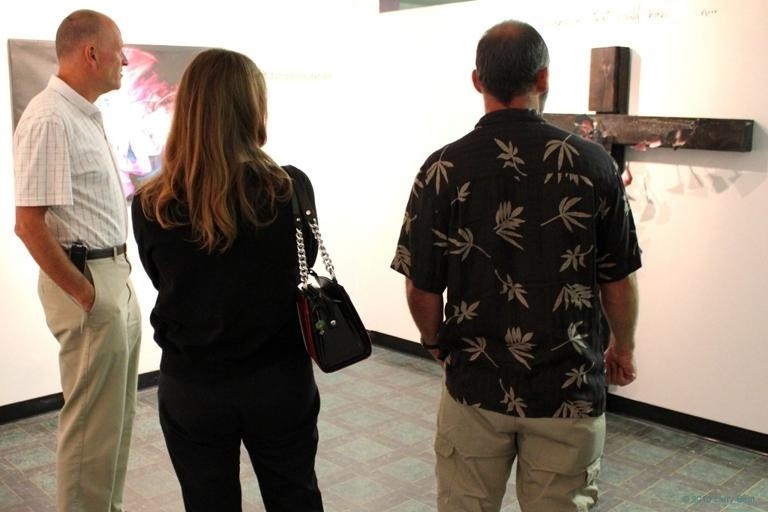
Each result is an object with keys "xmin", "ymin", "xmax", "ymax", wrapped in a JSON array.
[{"xmin": 298, "ymin": 274, "xmax": 372, "ymax": 373}]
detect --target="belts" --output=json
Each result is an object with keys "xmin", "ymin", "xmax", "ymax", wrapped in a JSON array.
[{"xmin": 64, "ymin": 244, "xmax": 124, "ymax": 259}]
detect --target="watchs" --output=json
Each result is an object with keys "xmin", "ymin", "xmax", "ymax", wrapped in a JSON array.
[{"xmin": 418, "ymin": 337, "xmax": 438, "ymax": 351}]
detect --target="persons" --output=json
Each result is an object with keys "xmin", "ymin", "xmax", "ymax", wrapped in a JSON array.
[
  {"xmin": 12, "ymin": 8, "xmax": 143, "ymax": 510},
  {"xmin": 389, "ymin": 18, "xmax": 643, "ymax": 511},
  {"xmin": 130, "ymin": 48, "xmax": 324, "ymax": 510}
]
